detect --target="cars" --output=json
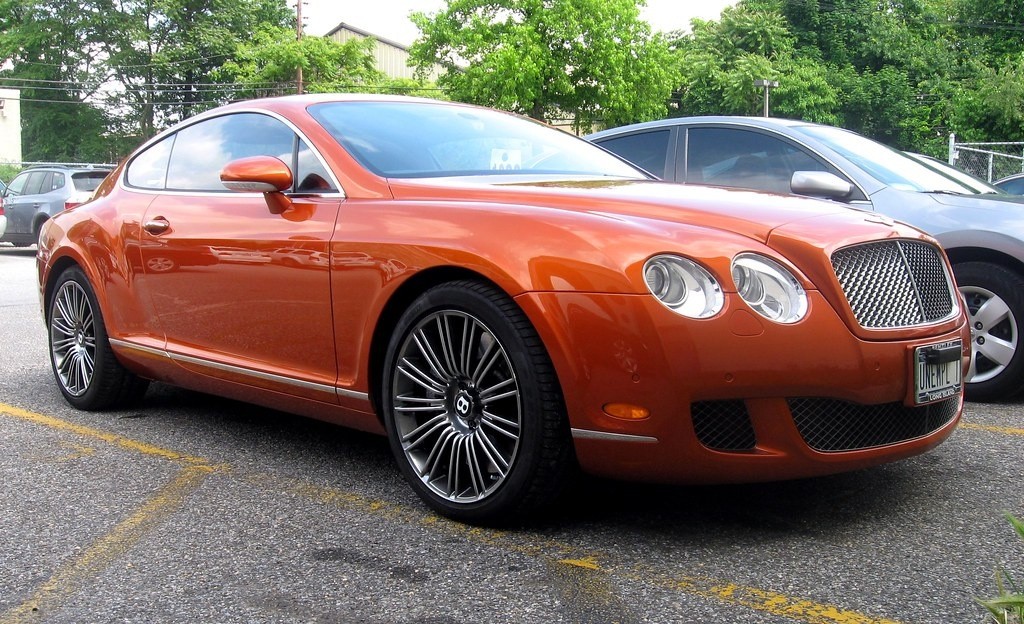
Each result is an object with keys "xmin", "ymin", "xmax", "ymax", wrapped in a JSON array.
[{"xmin": 512, "ymin": 114, "xmax": 1024, "ymax": 403}]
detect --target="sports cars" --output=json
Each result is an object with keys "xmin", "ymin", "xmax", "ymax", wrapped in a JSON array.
[{"xmin": 33, "ymin": 92, "xmax": 973, "ymax": 529}]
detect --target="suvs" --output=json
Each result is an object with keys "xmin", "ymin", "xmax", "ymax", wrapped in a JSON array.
[{"xmin": 0, "ymin": 163, "xmax": 142, "ymax": 253}]
